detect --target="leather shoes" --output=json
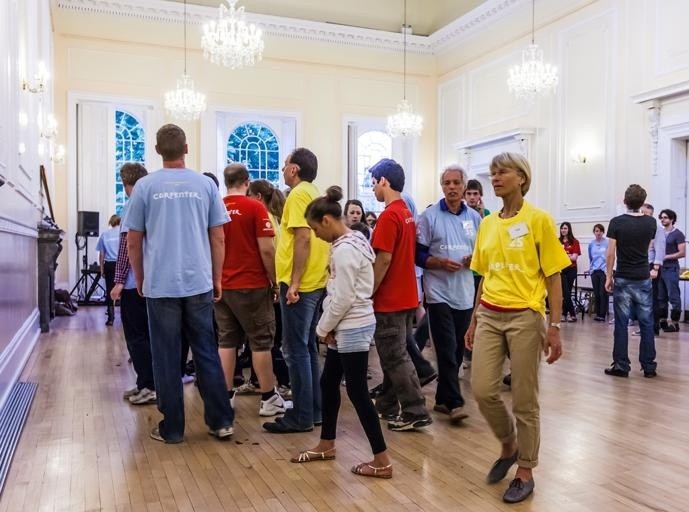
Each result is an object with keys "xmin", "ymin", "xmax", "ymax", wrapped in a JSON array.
[
  {"xmin": 502, "ymin": 474, "xmax": 534, "ymax": 504},
  {"xmin": 486, "ymin": 447, "xmax": 520, "ymax": 482}
]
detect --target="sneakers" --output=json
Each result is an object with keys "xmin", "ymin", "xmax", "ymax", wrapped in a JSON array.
[
  {"xmin": 182, "ymin": 374, "xmax": 196, "ymax": 384},
  {"xmin": 375, "ymin": 409, "xmax": 400, "ymax": 420},
  {"xmin": 122, "ymin": 388, "xmax": 139, "ymax": 399},
  {"xmin": 631, "ymin": 328, "xmax": 641, "ymax": 336},
  {"xmin": 567, "ymin": 315, "xmax": 577, "ymax": 322},
  {"xmin": 594, "ymin": 313, "xmax": 605, "ymax": 321},
  {"xmin": 129, "ymin": 387, "xmax": 158, "ymax": 405},
  {"xmin": 367, "ymin": 365, "xmax": 374, "ymax": 379},
  {"xmin": 628, "ymin": 318, "xmax": 635, "ymax": 326},
  {"xmin": 105, "ymin": 320, "xmax": 114, "ymax": 326},
  {"xmin": 609, "ymin": 317, "xmax": 615, "ymax": 324},
  {"xmin": 386, "ymin": 413, "xmax": 434, "ymax": 432},
  {"xmin": 229, "ymin": 393, "xmax": 237, "ymax": 412},
  {"xmin": 263, "ymin": 421, "xmax": 314, "ymax": 434},
  {"xmin": 434, "ymin": 403, "xmax": 451, "ymax": 416},
  {"xmin": 639, "ymin": 364, "xmax": 657, "ymax": 377},
  {"xmin": 560, "ymin": 315, "xmax": 565, "ymax": 322},
  {"xmin": 206, "ymin": 422, "xmax": 235, "ymax": 440},
  {"xmin": 450, "ymin": 407, "xmax": 470, "ymax": 421},
  {"xmin": 273, "ymin": 415, "xmax": 322, "ymax": 426},
  {"xmin": 502, "ymin": 373, "xmax": 511, "ymax": 387},
  {"xmin": 232, "ymin": 381, "xmax": 257, "ymax": 395},
  {"xmin": 368, "ymin": 384, "xmax": 386, "ymax": 397},
  {"xmin": 259, "ymin": 387, "xmax": 294, "ymax": 416},
  {"xmin": 419, "ymin": 371, "xmax": 438, "ymax": 388},
  {"xmin": 341, "ymin": 377, "xmax": 347, "ymax": 386},
  {"xmin": 664, "ymin": 322, "xmax": 680, "ymax": 333},
  {"xmin": 149, "ymin": 426, "xmax": 184, "ymax": 446},
  {"xmin": 604, "ymin": 363, "xmax": 629, "ymax": 378}
]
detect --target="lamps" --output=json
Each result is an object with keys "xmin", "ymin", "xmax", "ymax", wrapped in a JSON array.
[
  {"xmin": 20, "ymin": 60, "xmax": 48, "ymax": 96},
  {"xmin": 164, "ymin": 0, "xmax": 208, "ymax": 121},
  {"xmin": 566, "ymin": 127, "xmax": 602, "ymax": 172},
  {"xmin": 387, "ymin": 0, "xmax": 423, "ymax": 142},
  {"xmin": 507, "ymin": 0, "xmax": 560, "ymax": 99},
  {"xmin": 200, "ymin": 0, "xmax": 264, "ymax": 70}
]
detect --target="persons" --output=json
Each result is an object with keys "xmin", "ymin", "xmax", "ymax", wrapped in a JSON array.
[
  {"xmin": 202, "ymin": 171, "xmax": 219, "ymax": 191},
  {"xmin": 368, "ymin": 159, "xmax": 432, "ymax": 430},
  {"xmin": 123, "ymin": 123, "xmax": 235, "ymax": 444},
  {"xmin": 400, "ymin": 193, "xmax": 432, "ymax": 346},
  {"xmin": 111, "ymin": 162, "xmax": 157, "ymax": 406},
  {"xmin": 463, "ymin": 180, "xmax": 492, "ymax": 370},
  {"xmin": 239, "ymin": 343, "xmax": 252, "ymax": 368},
  {"xmin": 372, "ymin": 220, "xmax": 378, "ymax": 230},
  {"xmin": 290, "ymin": 186, "xmax": 392, "ymax": 479},
  {"xmin": 322, "ymin": 349, "xmax": 327, "ymax": 356},
  {"xmin": 656, "ymin": 209, "xmax": 686, "ymax": 332},
  {"xmin": 369, "ymin": 382, "xmax": 384, "ymax": 399},
  {"xmin": 605, "ymin": 184, "xmax": 657, "ymax": 378},
  {"xmin": 632, "ymin": 204, "xmax": 665, "ymax": 336},
  {"xmin": 414, "ymin": 311, "xmax": 430, "ymax": 351},
  {"xmin": 181, "ymin": 334, "xmax": 194, "ymax": 384},
  {"xmin": 340, "ymin": 223, "xmax": 373, "ymax": 386},
  {"xmin": 406, "ymin": 335, "xmax": 437, "ymax": 385},
  {"xmin": 365, "ymin": 211, "xmax": 376, "ymax": 226},
  {"xmin": 344, "ymin": 199, "xmax": 374, "ymax": 242},
  {"xmin": 588, "ymin": 224, "xmax": 610, "ymax": 321},
  {"xmin": 233, "ymin": 179, "xmax": 293, "ymax": 400},
  {"xmin": 263, "ymin": 148, "xmax": 331, "ymax": 433},
  {"xmin": 203, "ymin": 172, "xmax": 245, "ymax": 388},
  {"xmin": 415, "ymin": 162, "xmax": 483, "ymax": 424},
  {"xmin": 95, "ymin": 216, "xmax": 121, "ymax": 326},
  {"xmin": 215, "ymin": 162, "xmax": 293, "ymax": 416},
  {"xmin": 464, "ymin": 153, "xmax": 572, "ymax": 504},
  {"xmin": 503, "ymin": 349, "xmax": 512, "ymax": 384},
  {"xmin": 558, "ymin": 222, "xmax": 582, "ymax": 322}
]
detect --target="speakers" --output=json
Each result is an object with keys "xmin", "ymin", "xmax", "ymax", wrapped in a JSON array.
[{"xmin": 78, "ymin": 211, "xmax": 99, "ymax": 237}]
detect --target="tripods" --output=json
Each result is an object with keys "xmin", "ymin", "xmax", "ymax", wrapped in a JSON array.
[{"xmin": 69, "ymin": 237, "xmax": 106, "ymax": 305}]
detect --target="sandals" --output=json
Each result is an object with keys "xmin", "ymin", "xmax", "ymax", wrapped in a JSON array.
[
  {"xmin": 288, "ymin": 447, "xmax": 338, "ymax": 464},
  {"xmin": 349, "ymin": 463, "xmax": 398, "ymax": 480}
]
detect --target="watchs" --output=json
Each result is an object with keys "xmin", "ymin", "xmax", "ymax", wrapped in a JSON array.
[
  {"xmin": 653, "ymin": 267, "xmax": 659, "ymax": 272},
  {"xmin": 548, "ymin": 322, "xmax": 562, "ymax": 330}
]
